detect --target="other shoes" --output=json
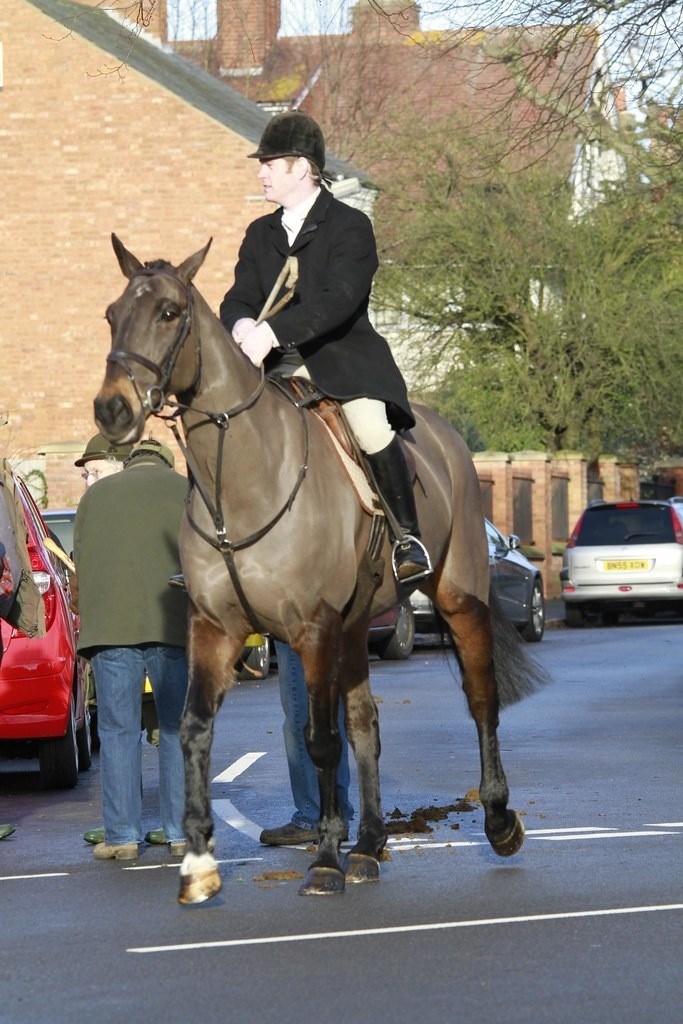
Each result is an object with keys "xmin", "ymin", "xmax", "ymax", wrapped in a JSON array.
[
  {"xmin": 94, "ymin": 840, "xmax": 139, "ymax": 860},
  {"xmin": 83, "ymin": 824, "xmax": 105, "ymax": 843},
  {"xmin": 0, "ymin": 823, "xmax": 17, "ymax": 839},
  {"xmin": 144, "ymin": 827, "xmax": 168, "ymax": 843},
  {"xmin": 170, "ymin": 838, "xmax": 216, "ymax": 855}
]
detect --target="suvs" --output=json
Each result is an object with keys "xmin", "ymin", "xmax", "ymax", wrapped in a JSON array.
[{"xmin": 559, "ymin": 495, "xmax": 683, "ymax": 629}]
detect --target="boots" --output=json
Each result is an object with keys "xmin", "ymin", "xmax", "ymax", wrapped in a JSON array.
[{"xmin": 359, "ymin": 432, "xmax": 427, "ymax": 579}]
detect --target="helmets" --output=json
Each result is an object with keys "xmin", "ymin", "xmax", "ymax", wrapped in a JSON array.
[
  {"xmin": 246, "ymin": 109, "xmax": 325, "ymax": 173},
  {"xmin": 74, "ymin": 431, "xmax": 134, "ymax": 467}
]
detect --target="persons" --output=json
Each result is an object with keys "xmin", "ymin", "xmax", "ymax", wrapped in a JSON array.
[
  {"xmin": 168, "ymin": 110, "xmax": 430, "ymax": 588},
  {"xmin": 73, "ymin": 434, "xmax": 216, "ymax": 858},
  {"xmin": 0, "ymin": 419, "xmax": 45, "ymax": 839}
]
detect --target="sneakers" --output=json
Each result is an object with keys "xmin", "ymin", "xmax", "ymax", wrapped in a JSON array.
[{"xmin": 260, "ymin": 822, "xmax": 350, "ymax": 843}]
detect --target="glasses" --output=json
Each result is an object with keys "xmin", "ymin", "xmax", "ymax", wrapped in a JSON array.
[{"xmin": 81, "ymin": 465, "xmax": 117, "ymax": 482}]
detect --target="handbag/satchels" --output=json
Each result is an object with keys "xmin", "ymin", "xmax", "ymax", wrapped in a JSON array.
[{"xmin": 4, "ymin": 571, "xmax": 46, "ymax": 639}]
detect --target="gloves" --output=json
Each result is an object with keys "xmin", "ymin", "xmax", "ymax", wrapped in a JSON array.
[
  {"xmin": 232, "ymin": 318, "xmax": 256, "ymax": 347},
  {"xmin": 240, "ymin": 320, "xmax": 280, "ymax": 368}
]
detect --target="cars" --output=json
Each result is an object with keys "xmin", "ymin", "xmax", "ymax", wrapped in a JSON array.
[
  {"xmin": 408, "ymin": 515, "xmax": 547, "ymax": 643},
  {"xmin": 37, "ymin": 509, "xmax": 417, "ymax": 717},
  {"xmin": 0, "ymin": 452, "xmax": 94, "ymax": 789}
]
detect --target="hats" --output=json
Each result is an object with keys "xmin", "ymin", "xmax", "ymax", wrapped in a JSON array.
[{"xmin": 129, "ymin": 439, "xmax": 174, "ymax": 470}]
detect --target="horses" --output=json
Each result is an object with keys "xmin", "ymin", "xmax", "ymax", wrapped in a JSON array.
[{"xmin": 94, "ymin": 231, "xmax": 552, "ymax": 907}]
